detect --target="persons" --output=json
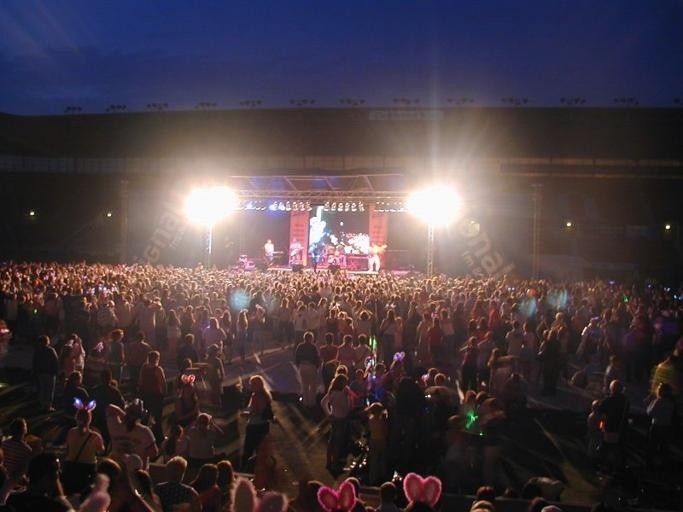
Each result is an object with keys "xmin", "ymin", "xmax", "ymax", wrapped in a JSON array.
[
  {"xmin": 368, "ymin": 241, "xmax": 384, "ymax": 273},
  {"xmin": 290, "ymin": 238, "xmax": 300, "ymax": 265},
  {"xmin": 311, "ymin": 243, "xmax": 321, "ymax": 273},
  {"xmin": 264, "ymin": 238, "xmax": 274, "ymax": 267}
]
